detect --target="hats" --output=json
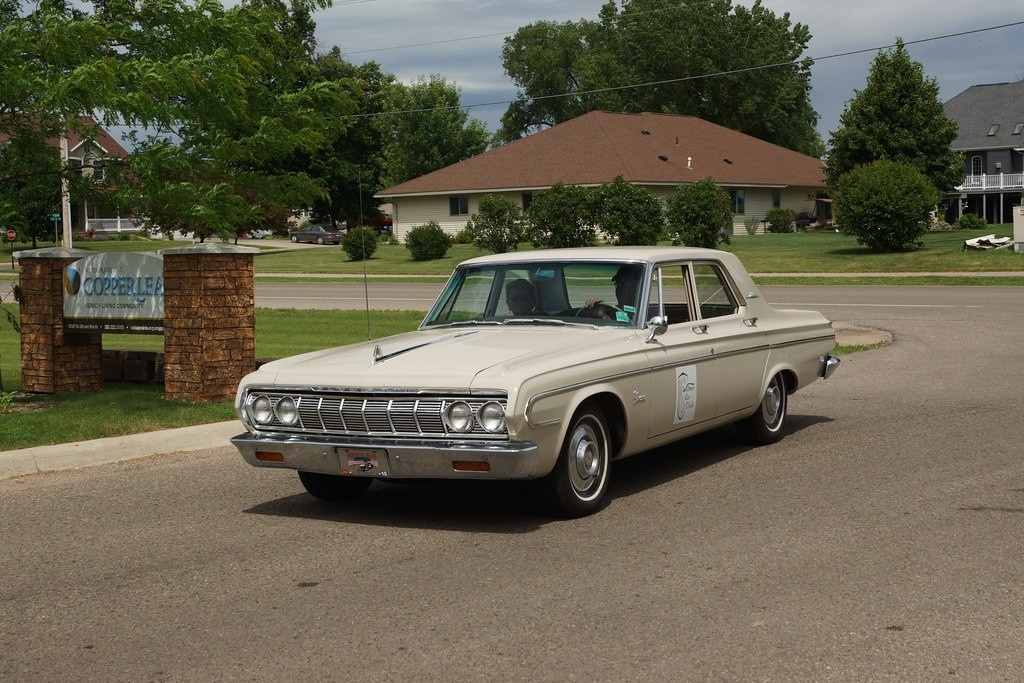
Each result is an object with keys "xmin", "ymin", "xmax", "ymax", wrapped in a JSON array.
[{"xmin": 611, "ymin": 265, "xmax": 642, "ymax": 282}]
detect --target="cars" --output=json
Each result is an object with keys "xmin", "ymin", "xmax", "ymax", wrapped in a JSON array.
[
  {"xmin": 289, "ymin": 225, "xmax": 345, "ymax": 245},
  {"xmin": 229, "ymin": 244, "xmax": 842, "ymax": 520}
]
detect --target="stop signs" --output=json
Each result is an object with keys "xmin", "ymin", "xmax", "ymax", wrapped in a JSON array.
[{"xmin": 7, "ymin": 230, "xmax": 16, "ymax": 241}]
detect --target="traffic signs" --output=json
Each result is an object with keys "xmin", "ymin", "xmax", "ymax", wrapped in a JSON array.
[{"xmin": 50, "ymin": 214, "xmax": 61, "ymax": 221}]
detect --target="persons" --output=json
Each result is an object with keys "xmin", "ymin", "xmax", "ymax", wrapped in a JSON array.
[
  {"xmin": 585, "ymin": 264, "xmax": 643, "ymax": 322},
  {"xmin": 503, "ymin": 280, "xmax": 535, "ymax": 315}
]
